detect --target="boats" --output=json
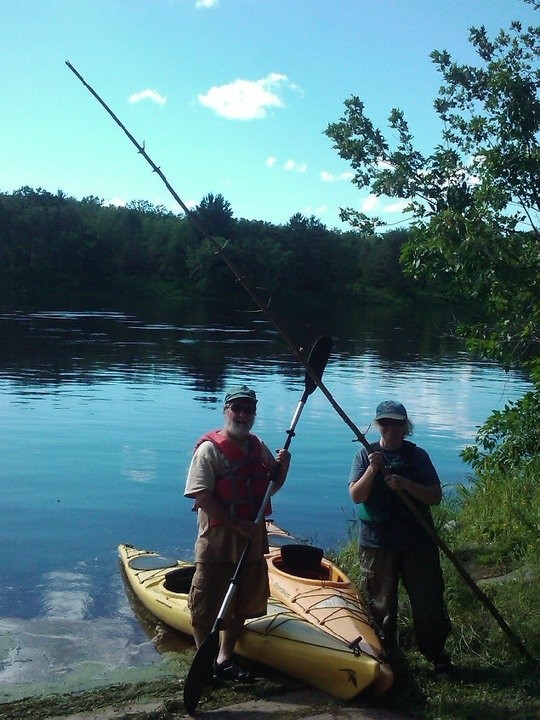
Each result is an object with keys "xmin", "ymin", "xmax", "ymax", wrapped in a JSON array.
[
  {"xmin": 263, "ymin": 519, "xmax": 393, "ymax": 701},
  {"xmin": 116, "ymin": 543, "xmax": 380, "ymax": 703}
]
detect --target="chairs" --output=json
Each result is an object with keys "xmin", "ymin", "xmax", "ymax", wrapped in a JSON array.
[
  {"xmin": 164, "ymin": 566, "xmax": 196, "ymax": 594},
  {"xmin": 279, "ymin": 545, "xmax": 324, "ymax": 580}
]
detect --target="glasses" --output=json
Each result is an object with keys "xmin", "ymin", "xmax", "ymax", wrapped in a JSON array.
[
  {"xmin": 228, "ymin": 405, "xmax": 254, "ymax": 414},
  {"xmin": 378, "ymin": 420, "xmax": 402, "ymax": 427}
]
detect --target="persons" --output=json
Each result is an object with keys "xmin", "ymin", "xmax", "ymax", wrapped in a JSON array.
[
  {"xmin": 348, "ymin": 400, "xmax": 469, "ymax": 687},
  {"xmin": 183, "ymin": 384, "xmax": 291, "ymax": 689}
]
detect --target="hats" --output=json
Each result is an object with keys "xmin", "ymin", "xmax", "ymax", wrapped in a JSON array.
[
  {"xmin": 224, "ymin": 385, "xmax": 258, "ymax": 404},
  {"xmin": 375, "ymin": 401, "xmax": 407, "ymax": 420}
]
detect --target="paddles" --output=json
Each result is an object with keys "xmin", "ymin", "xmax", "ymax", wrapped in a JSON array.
[{"xmin": 184, "ymin": 336, "xmax": 333, "ymax": 714}]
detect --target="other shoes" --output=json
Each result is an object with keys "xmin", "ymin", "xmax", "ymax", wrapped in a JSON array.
[
  {"xmin": 214, "ymin": 658, "xmax": 254, "ymax": 684},
  {"xmin": 435, "ymin": 660, "xmax": 460, "ymax": 680},
  {"xmin": 210, "ymin": 669, "xmax": 227, "ymax": 689}
]
